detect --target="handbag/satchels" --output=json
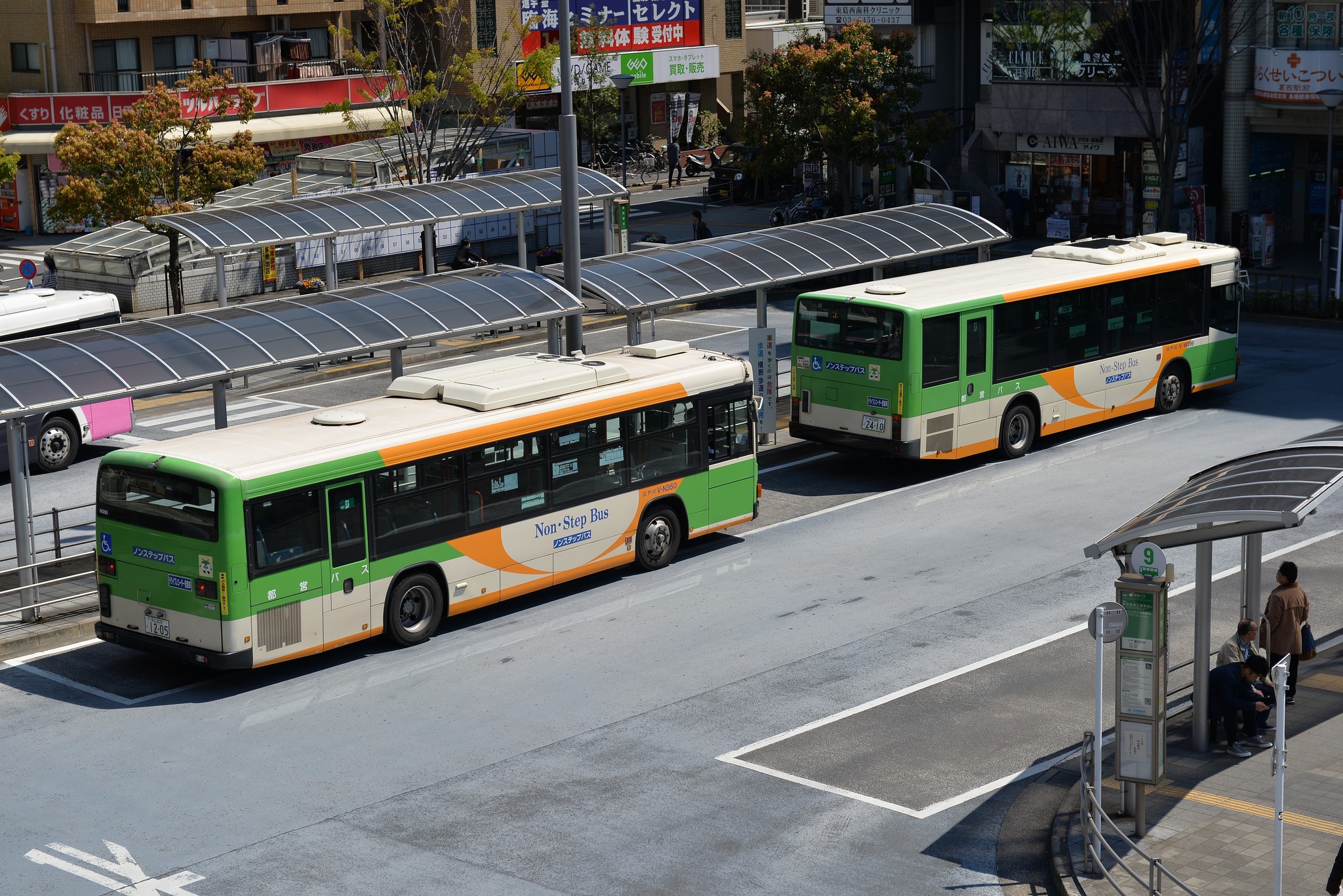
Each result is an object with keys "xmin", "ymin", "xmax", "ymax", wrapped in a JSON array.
[{"xmin": 1298, "ymin": 620, "xmax": 1317, "ymax": 661}]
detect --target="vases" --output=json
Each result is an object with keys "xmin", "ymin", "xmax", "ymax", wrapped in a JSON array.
[
  {"xmin": 537, "ymin": 255, "xmax": 562, "ymax": 266},
  {"xmin": 299, "ymin": 285, "xmax": 325, "ymax": 295}
]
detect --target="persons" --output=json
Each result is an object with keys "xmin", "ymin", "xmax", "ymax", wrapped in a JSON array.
[
  {"xmin": 1189, "ymin": 654, "xmax": 1273, "ymax": 757},
  {"xmin": 692, "ymin": 211, "xmax": 713, "ymax": 241},
  {"xmin": 1259, "ymin": 561, "xmax": 1309, "ymax": 704},
  {"xmin": 1216, "ymin": 618, "xmax": 1276, "ymax": 738},
  {"xmin": 667, "ymin": 136, "xmax": 683, "ymax": 189}
]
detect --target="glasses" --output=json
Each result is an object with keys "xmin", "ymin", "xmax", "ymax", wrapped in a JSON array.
[
  {"xmin": 43, "ymin": 260, "xmax": 45, "ymax": 263},
  {"xmin": 1249, "ymin": 628, "xmax": 1258, "ymax": 631}
]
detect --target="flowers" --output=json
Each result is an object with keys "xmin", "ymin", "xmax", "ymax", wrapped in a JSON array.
[
  {"xmin": 536, "ymin": 247, "xmax": 563, "ymax": 257},
  {"xmin": 296, "ymin": 277, "xmax": 323, "ymax": 290},
  {"xmin": 641, "ymin": 234, "xmax": 667, "ymax": 243}
]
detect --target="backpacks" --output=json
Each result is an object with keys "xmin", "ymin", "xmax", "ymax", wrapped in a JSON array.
[{"xmin": 699, "ymin": 221, "xmax": 713, "ymax": 239}]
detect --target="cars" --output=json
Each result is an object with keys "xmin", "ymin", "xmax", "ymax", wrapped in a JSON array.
[
  {"xmin": 707, "ymin": 140, "xmax": 802, "ymax": 206},
  {"xmin": 587, "ymin": 133, "xmax": 669, "ymax": 186},
  {"xmin": 1, "ymin": 397, "xmax": 136, "ymax": 472}
]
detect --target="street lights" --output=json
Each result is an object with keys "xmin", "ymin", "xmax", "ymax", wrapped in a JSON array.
[{"xmin": 609, "ymin": 74, "xmax": 636, "ymax": 200}]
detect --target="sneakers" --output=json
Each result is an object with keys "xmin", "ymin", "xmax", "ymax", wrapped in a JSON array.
[
  {"xmin": 1285, "ymin": 696, "xmax": 1295, "ymax": 705},
  {"xmin": 676, "ymin": 182, "xmax": 683, "ymax": 187},
  {"xmin": 1226, "ymin": 741, "xmax": 1252, "ymax": 757},
  {"xmin": 669, "ymin": 185, "xmax": 675, "ymax": 188},
  {"xmin": 1245, "ymin": 735, "xmax": 1273, "ymax": 748},
  {"xmin": 1257, "ymin": 722, "xmax": 1276, "ymax": 737}
]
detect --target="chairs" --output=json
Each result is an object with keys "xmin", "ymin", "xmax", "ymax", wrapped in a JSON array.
[
  {"xmin": 341, "ymin": 502, "xmax": 440, "ymax": 540},
  {"xmin": 254, "ymin": 525, "xmax": 302, "ymax": 570}
]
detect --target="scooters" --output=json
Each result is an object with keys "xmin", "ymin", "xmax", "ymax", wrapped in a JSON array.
[{"xmin": 682, "ymin": 140, "xmax": 724, "ymax": 177}]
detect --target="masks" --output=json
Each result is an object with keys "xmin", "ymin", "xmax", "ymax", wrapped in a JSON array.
[{"xmin": 773, "ymin": 217, "xmax": 777, "ymax": 221}]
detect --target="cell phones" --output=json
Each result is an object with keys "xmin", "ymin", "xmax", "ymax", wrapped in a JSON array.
[{"xmin": 1264, "ymin": 703, "xmax": 1272, "ymax": 707}]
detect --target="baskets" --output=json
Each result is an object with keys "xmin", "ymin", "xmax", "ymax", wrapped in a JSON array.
[
  {"xmin": 643, "ymin": 158, "xmax": 655, "ymax": 166},
  {"xmin": 595, "ymin": 149, "xmax": 602, "ymax": 155},
  {"xmin": 645, "ymin": 137, "xmax": 656, "ymax": 145},
  {"xmin": 601, "ymin": 145, "xmax": 615, "ymax": 155},
  {"xmin": 792, "ymin": 195, "xmax": 806, "ymax": 204},
  {"xmin": 854, "ymin": 202, "xmax": 868, "ymax": 211},
  {"xmin": 812, "ymin": 173, "xmax": 826, "ymax": 181}
]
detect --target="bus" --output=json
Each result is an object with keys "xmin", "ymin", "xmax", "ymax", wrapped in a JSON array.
[
  {"xmin": 788, "ymin": 232, "xmax": 1251, "ymax": 461},
  {"xmin": 92, "ymin": 338, "xmax": 767, "ymax": 670}
]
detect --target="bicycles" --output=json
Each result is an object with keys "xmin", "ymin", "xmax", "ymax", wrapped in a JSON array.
[{"xmin": 769, "ymin": 170, "xmax": 874, "ymax": 228}]
